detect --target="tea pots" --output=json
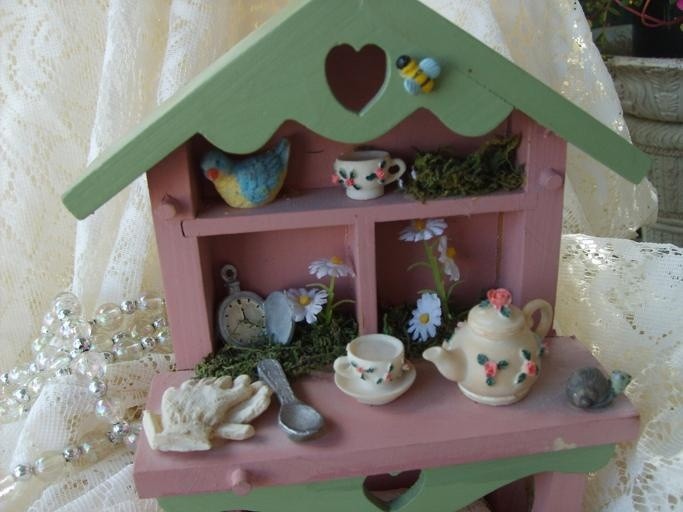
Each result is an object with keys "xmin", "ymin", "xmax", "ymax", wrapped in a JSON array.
[{"xmin": 422, "ymin": 287, "xmax": 554, "ymax": 407}]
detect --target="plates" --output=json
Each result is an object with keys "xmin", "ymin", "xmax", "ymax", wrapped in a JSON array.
[{"xmin": 334, "ymin": 360, "xmax": 416, "ymax": 404}]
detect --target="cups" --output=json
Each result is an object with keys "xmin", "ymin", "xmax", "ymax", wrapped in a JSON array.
[
  {"xmin": 333, "ymin": 333, "xmax": 405, "ymax": 389},
  {"xmin": 330, "ymin": 151, "xmax": 406, "ymax": 201}
]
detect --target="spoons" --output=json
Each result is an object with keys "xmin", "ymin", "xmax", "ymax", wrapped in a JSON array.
[{"xmin": 256, "ymin": 357, "xmax": 325, "ymax": 441}]
